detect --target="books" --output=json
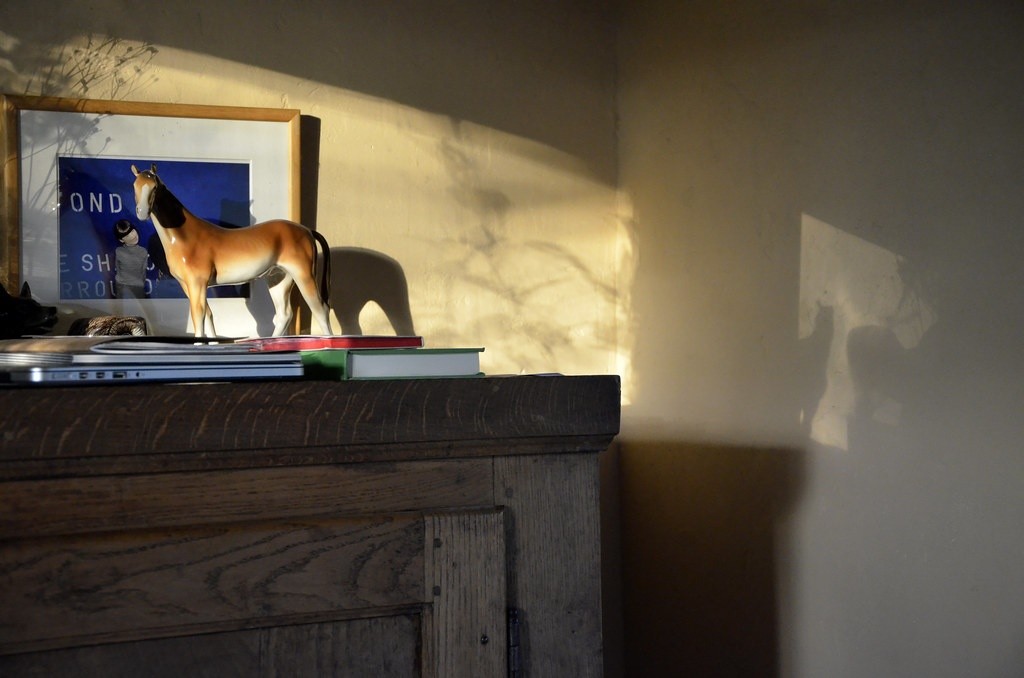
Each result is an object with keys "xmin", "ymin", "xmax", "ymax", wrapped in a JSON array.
[{"xmin": 0, "ymin": 331, "xmax": 488, "ymax": 384}]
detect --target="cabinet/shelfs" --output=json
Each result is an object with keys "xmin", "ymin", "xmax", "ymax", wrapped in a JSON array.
[{"xmin": 0, "ymin": 374, "xmax": 621, "ymax": 678}]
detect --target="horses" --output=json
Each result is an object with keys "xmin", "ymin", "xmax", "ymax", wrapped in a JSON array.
[{"xmin": 131, "ymin": 163, "xmax": 334, "ymax": 346}]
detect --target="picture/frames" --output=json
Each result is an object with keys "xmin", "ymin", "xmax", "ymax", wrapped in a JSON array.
[{"xmin": 0, "ymin": 95, "xmax": 301, "ymax": 337}]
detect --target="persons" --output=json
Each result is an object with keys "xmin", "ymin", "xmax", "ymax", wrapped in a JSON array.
[{"xmin": 111, "ymin": 220, "xmax": 153, "ymax": 298}]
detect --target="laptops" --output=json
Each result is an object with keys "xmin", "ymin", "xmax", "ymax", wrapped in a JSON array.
[{"xmin": 11, "ymin": 363, "xmax": 304, "ymax": 383}]
